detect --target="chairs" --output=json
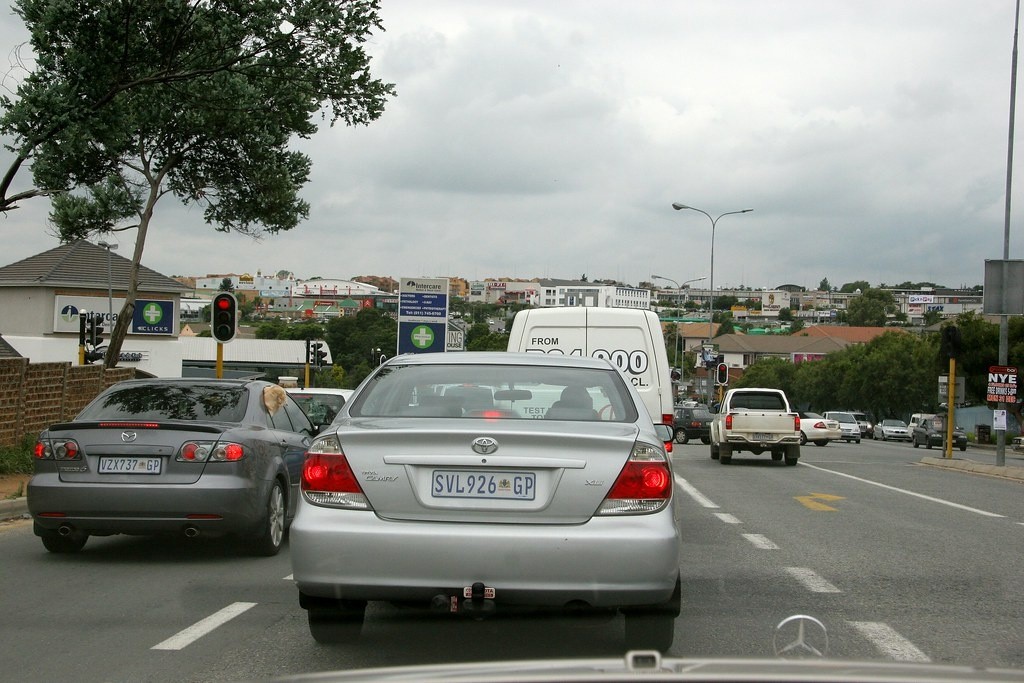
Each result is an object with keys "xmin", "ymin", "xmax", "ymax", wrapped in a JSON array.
[{"xmin": 397, "ymin": 396, "xmax": 601, "ymax": 421}]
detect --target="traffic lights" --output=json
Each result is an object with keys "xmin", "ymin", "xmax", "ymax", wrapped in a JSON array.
[
  {"xmin": 88, "ymin": 311, "xmax": 106, "ymax": 348},
  {"xmin": 717, "ymin": 363, "xmax": 727, "ymax": 383},
  {"xmin": 210, "ymin": 290, "xmax": 241, "ymax": 341}
]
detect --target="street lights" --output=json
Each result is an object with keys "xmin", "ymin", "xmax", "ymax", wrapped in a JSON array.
[
  {"xmin": 651, "ymin": 274, "xmax": 709, "ymax": 351},
  {"xmin": 671, "ymin": 202, "xmax": 756, "ymax": 340},
  {"xmin": 98, "ymin": 240, "xmax": 119, "ymax": 341}
]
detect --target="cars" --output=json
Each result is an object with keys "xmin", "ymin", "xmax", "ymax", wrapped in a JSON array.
[
  {"xmin": 26, "ymin": 376, "xmax": 332, "ymax": 557},
  {"xmin": 821, "ymin": 411, "xmax": 862, "ymax": 444},
  {"xmin": 283, "ymin": 385, "xmax": 357, "ymax": 426},
  {"xmin": 911, "ymin": 415, "xmax": 969, "ymax": 451},
  {"xmin": 847, "ymin": 412, "xmax": 874, "ymax": 439},
  {"xmin": 907, "ymin": 413, "xmax": 936, "ymax": 437},
  {"xmin": 291, "ymin": 352, "xmax": 685, "ymax": 653},
  {"xmin": 870, "ymin": 420, "xmax": 911, "ymax": 443},
  {"xmin": 800, "ymin": 412, "xmax": 843, "ymax": 447},
  {"xmin": 672, "ymin": 401, "xmax": 712, "ymax": 445}
]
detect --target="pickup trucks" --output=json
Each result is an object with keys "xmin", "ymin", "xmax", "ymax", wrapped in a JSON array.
[{"xmin": 709, "ymin": 387, "xmax": 802, "ymax": 465}]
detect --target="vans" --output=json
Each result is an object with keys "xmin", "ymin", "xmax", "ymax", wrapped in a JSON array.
[{"xmin": 504, "ymin": 305, "xmax": 678, "ymax": 464}]
